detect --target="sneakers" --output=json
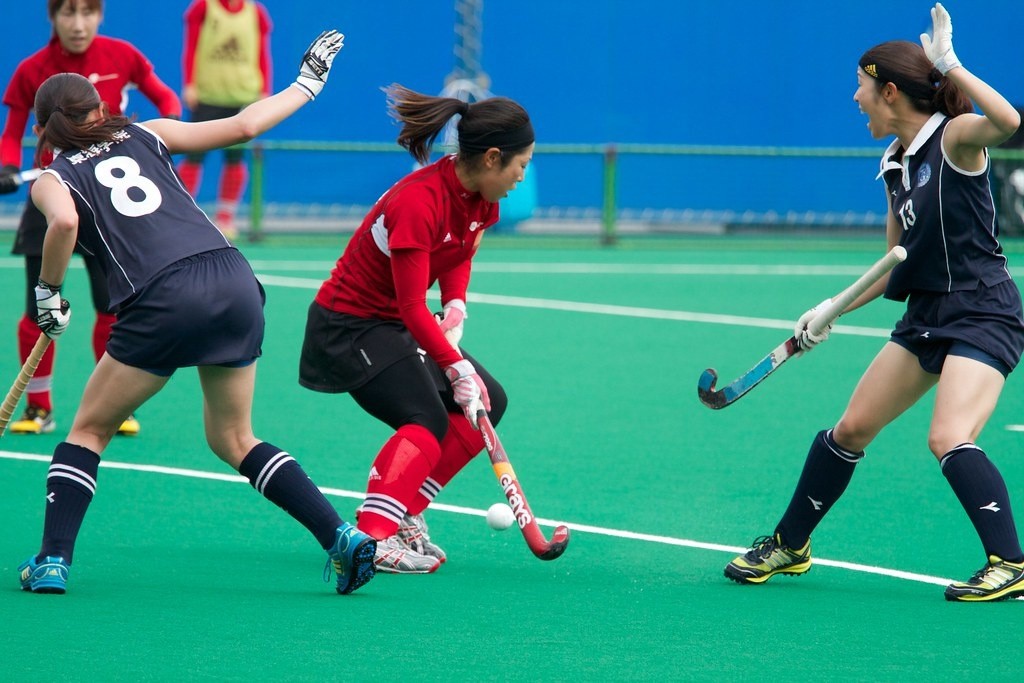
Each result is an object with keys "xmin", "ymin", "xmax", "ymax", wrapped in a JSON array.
[
  {"xmin": 944, "ymin": 552, "xmax": 1024, "ymax": 602},
  {"xmin": 725, "ymin": 528, "xmax": 812, "ymax": 585},
  {"xmin": 119, "ymin": 415, "xmax": 138, "ymax": 436},
  {"xmin": 11, "ymin": 401, "xmax": 55, "ymax": 432},
  {"xmin": 18, "ymin": 552, "xmax": 71, "ymax": 594},
  {"xmin": 323, "ymin": 523, "xmax": 377, "ymax": 594},
  {"xmin": 400, "ymin": 512, "xmax": 446, "ymax": 563},
  {"xmin": 373, "ymin": 535, "xmax": 440, "ymax": 574}
]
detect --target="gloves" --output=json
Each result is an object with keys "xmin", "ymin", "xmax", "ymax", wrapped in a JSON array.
[
  {"xmin": 919, "ymin": 3, "xmax": 963, "ymax": 77},
  {"xmin": 794, "ymin": 297, "xmax": 841, "ymax": 351},
  {"xmin": 444, "ymin": 358, "xmax": 490, "ymax": 430},
  {"xmin": 435, "ymin": 299, "xmax": 467, "ymax": 350},
  {"xmin": 293, "ymin": 30, "xmax": 347, "ymax": 102},
  {"xmin": 34, "ymin": 279, "xmax": 71, "ymax": 340},
  {"xmin": 0, "ymin": 165, "xmax": 19, "ymax": 194}
]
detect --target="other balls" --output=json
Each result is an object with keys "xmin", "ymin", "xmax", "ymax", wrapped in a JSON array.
[{"xmin": 487, "ymin": 502, "xmax": 515, "ymax": 531}]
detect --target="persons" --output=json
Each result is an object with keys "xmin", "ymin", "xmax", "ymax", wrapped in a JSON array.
[
  {"xmin": 0, "ymin": 0, "xmax": 182, "ymax": 436},
  {"xmin": 723, "ymin": 2, "xmax": 1024, "ymax": 603},
  {"xmin": 19, "ymin": 30, "xmax": 376, "ymax": 593},
  {"xmin": 299, "ymin": 84, "xmax": 536, "ymax": 573},
  {"xmin": 178, "ymin": 0, "xmax": 271, "ymax": 240}
]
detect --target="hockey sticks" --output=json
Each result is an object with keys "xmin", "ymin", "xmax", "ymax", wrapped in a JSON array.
[
  {"xmin": 432, "ymin": 310, "xmax": 571, "ymax": 560},
  {"xmin": 0, "ymin": 297, "xmax": 71, "ymax": 439},
  {"xmin": 13, "ymin": 165, "xmax": 50, "ymax": 185},
  {"xmin": 695, "ymin": 243, "xmax": 907, "ymax": 410}
]
